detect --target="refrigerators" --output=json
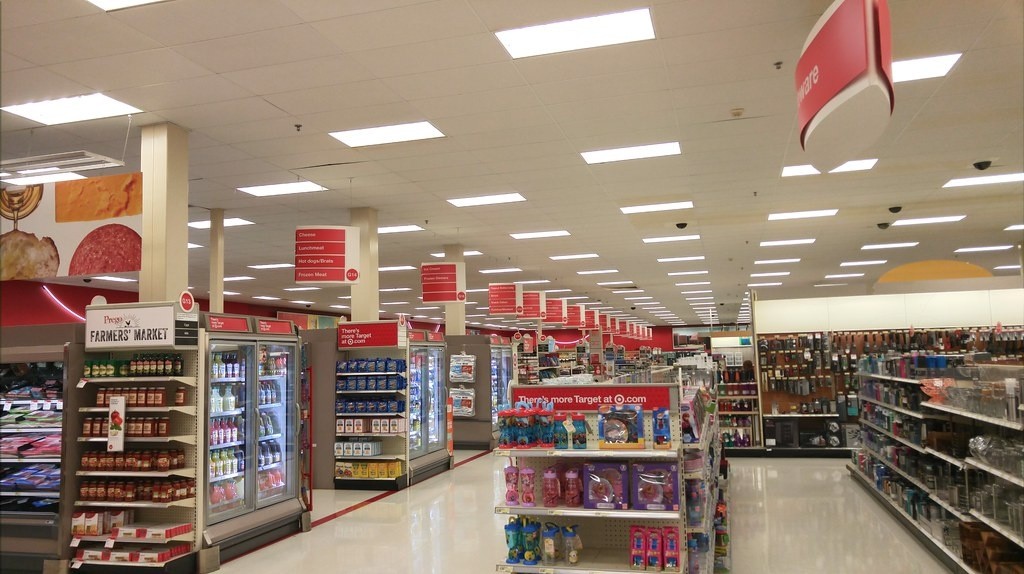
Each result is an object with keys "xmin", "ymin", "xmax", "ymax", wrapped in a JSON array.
[
  {"xmin": 199, "ymin": 311, "xmax": 299, "ymax": 565},
  {"xmin": 490, "ymin": 344, "xmax": 512, "ymax": 432},
  {"xmin": 406, "ymin": 328, "xmax": 451, "ymax": 485}
]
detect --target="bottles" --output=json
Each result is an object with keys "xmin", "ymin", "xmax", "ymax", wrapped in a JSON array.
[
  {"xmin": 848, "ymin": 348, "xmax": 966, "ymax": 523},
  {"xmin": 500, "ymin": 401, "xmax": 583, "ymax": 568},
  {"xmin": 211, "ymin": 351, "xmax": 283, "ymax": 503},
  {"xmin": 80, "ymin": 354, "xmax": 194, "ymax": 503}
]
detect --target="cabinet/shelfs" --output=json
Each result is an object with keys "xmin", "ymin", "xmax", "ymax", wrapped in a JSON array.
[
  {"xmin": 0, "ymin": 300, "xmax": 204, "ymax": 574},
  {"xmin": 333, "ymin": 357, "xmax": 409, "ymax": 492},
  {"xmin": 717, "ymin": 381, "xmax": 766, "ymax": 458},
  {"xmin": 845, "ymin": 370, "xmax": 1024, "ymax": 574},
  {"xmin": 517, "ymin": 350, "xmax": 576, "ymax": 383},
  {"xmin": 493, "ymin": 364, "xmax": 731, "ymax": 574}
]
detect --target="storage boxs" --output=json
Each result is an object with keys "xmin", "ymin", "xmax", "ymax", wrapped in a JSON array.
[
  {"xmin": 598, "ymin": 404, "xmax": 645, "ymax": 450},
  {"xmin": 683, "ymin": 386, "xmax": 706, "ymax": 444},
  {"xmin": 334, "ymin": 358, "xmax": 406, "ymax": 479},
  {"xmin": 646, "ymin": 527, "xmax": 662, "ymax": 571},
  {"xmin": 583, "ymin": 460, "xmax": 630, "ymax": 510},
  {"xmin": 652, "ymin": 406, "xmax": 671, "ymax": 450},
  {"xmin": 629, "ymin": 525, "xmax": 646, "ymax": 570},
  {"xmin": 662, "ymin": 526, "xmax": 680, "ymax": 572},
  {"xmin": 632, "ymin": 461, "xmax": 681, "ymax": 511},
  {"xmin": 71, "ymin": 510, "xmax": 192, "ymax": 564}
]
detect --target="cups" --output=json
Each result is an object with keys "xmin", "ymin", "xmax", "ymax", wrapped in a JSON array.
[
  {"xmin": 968, "ymin": 436, "xmax": 1024, "ymax": 542},
  {"xmin": 948, "ymin": 382, "xmax": 1024, "ymax": 424}
]
